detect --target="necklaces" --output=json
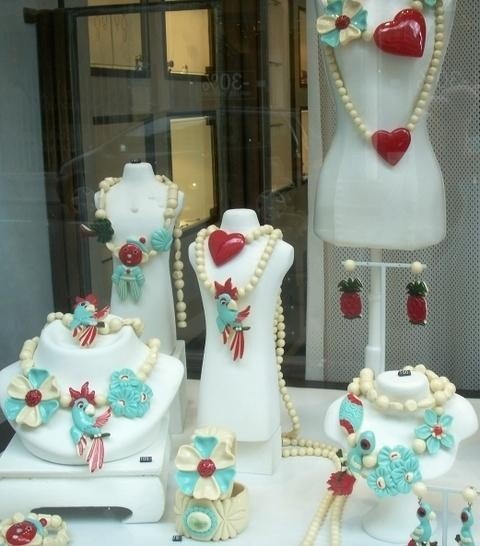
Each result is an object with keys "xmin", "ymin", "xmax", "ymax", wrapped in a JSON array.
[
  {"xmin": 0, "ymin": 295, "xmax": 160, "ymax": 472},
  {"xmin": 311, "ymin": 0, "xmax": 444, "ymax": 165},
  {"xmin": 1, "ymin": 513, "xmax": 69, "ymax": 546},
  {"xmin": 283, "ymin": 439, "xmax": 356, "ymax": 546},
  {"xmin": 81, "ymin": 172, "xmax": 188, "ymax": 328},
  {"xmin": 340, "ymin": 363, "xmax": 458, "ymax": 498},
  {"xmin": 195, "ymin": 222, "xmax": 301, "ymax": 437}
]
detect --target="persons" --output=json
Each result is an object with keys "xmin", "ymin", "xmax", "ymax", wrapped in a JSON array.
[
  {"xmin": 189, "ymin": 208, "xmax": 295, "ymax": 443},
  {"xmin": 94, "ymin": 160, "xmax": 184, "ymax": 356}
]
